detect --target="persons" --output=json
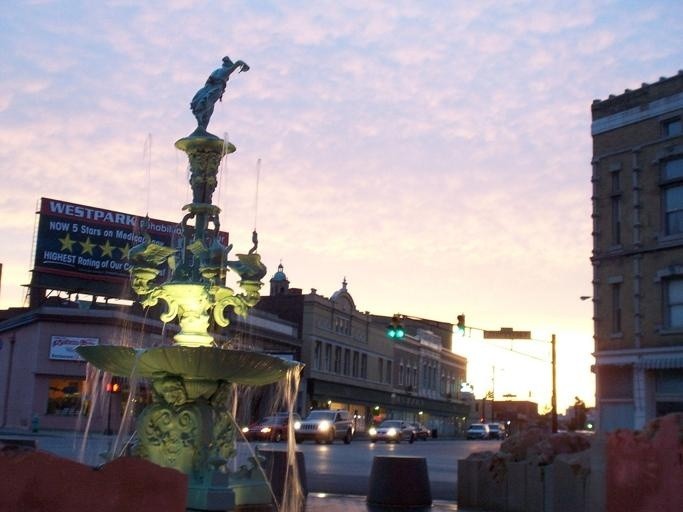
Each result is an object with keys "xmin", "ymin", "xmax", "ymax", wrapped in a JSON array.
[{"xmin": 190, "ymin": 56, "xmax": 249, "ymax": 128}]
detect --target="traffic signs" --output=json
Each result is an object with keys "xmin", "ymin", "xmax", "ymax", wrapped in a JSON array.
[{"xmin": 481, "ymin": 330, "xmax": 531, "ymax": 340}]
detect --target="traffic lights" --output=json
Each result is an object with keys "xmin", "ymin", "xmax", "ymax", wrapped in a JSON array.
[
  {"xmin": 455, "ymin": 313, "xmax": 466, "ymax": 338},
  {"xmin": 395, "ymin": 318, "xmax": 406, "ymax": 338},
  {"xmin": 105, "ymin": 384, "xmax": 120, "ymax": 393},
  {"xmin": 387, "ymin": 316, "xmax": 395, "ymax": 338}
]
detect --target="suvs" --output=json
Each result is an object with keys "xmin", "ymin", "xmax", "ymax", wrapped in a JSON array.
[{"xmin": 292, "ymin": 407, "xmax": 353, "ymax": 446}]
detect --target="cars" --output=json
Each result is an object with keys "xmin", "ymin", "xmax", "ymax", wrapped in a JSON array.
[
  {"xmin": 238, "ymin": 412, "xmax": 302, "ymax": 443},
  {"xmin": 366, "ymin": 418, "xmax": 512, "ymax": 443}
]
